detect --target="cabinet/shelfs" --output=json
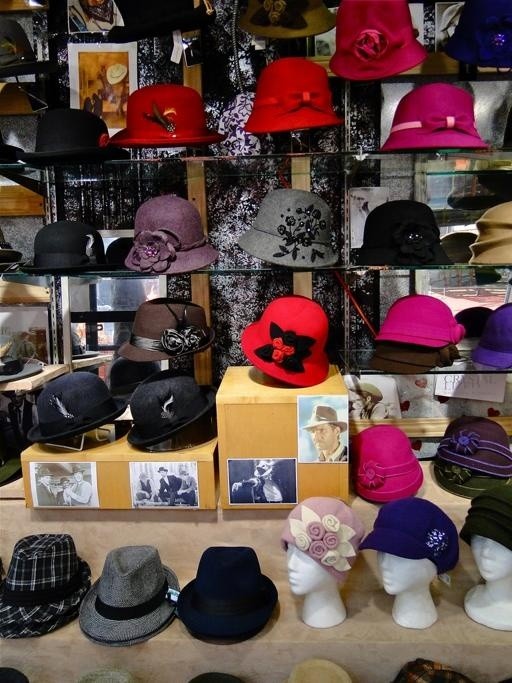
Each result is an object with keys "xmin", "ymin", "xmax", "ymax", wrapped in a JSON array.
[
  {"xmin": 1, "ymin": 0, "xmax": 512, "ymax": 445},
  {"xmin": 216, "ymin": 365, "xmax": 349, "ymax": 512}
]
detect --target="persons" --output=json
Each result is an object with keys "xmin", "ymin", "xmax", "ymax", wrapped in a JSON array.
[
  {"xmin": 281, "ymin": 496, "xmax": 364, "ymax": 628},
  {"xmin": 350, "ymin": 383, "xmax": 395, "ymax": 419},
  {"xmin": 35, "ymin": 463, "xmax": 94, "ymax": 505},
  {"xmin": 231, "ymin": 460, "xmax": 296, "ymax": 504},
  {"xmin": 133, "ymin": 465, "xmax": 197, "ymax": 506},
  {"xmin": 459, "ymin": 485, "xmax": 511, "ymax": 633},
  {"xmin": 302, "ymin": 406, "xmax": 350, "ymax": 463},
  {"xmin": 357, "ymin": 497, "xmax": 460, "ymax": 629}
]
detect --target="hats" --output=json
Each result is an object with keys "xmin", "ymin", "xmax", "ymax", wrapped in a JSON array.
[
  {"xmin": 2, "ymin": 0, "xmax": 512, "ymax": 170},
  {"xmin": 108, "ymin": 297, "xmax": 217, "ymax": 445},
  {"xmin": 471, "ymin": 302, "xmax": 512, "ymax": 368},
  {"xmin": 36, "ymin": 468, "xmax": 53, "ymax": 477},
  {"xmin": 26, "ymin": 372, "xmax": 128, "ymax": 443},
  {"xmin": 241, "ymin": 295, "xmax": 328, "ymax": 387},
  {"xmin": 349, "ymin": 383, "xmax": 383, "ymax": 403},
  {"xmin": 69, "ymin": 464, "xmax": 85, "ymax": 476},
  {"xmin": 280, "ymin": 416, "xmax": 512, "ymax": 587},
  {"xmin": 0, "ymin": 192, "xmax": 511, "ymax": 280},
  {"xmin": 370, "ymin": 294, "xmax": 465, "ymax": 374},
  {"xmin": 157, "ymin": 467, "xmax": 168, "ymax": 472},
  {"xmin": 303, "ymin": 406, "xmax": 347, "ymax": 431},
  {"xmin": 0, "ymin": 534, "xmax": 278, "ymax": 645},
  {"xmin": 60, "ymin": 477, "xmax": 69, "ymax": 485}
]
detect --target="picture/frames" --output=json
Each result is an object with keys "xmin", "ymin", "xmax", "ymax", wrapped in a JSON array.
[{"xmin": 67, "ymin": 42, "xmax": 137, "ymax": 139}]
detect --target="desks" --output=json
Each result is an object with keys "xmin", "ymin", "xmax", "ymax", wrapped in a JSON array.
[{"xmin": 0, "ymin": 462, "xmax": 512, "ymax": 682}]
